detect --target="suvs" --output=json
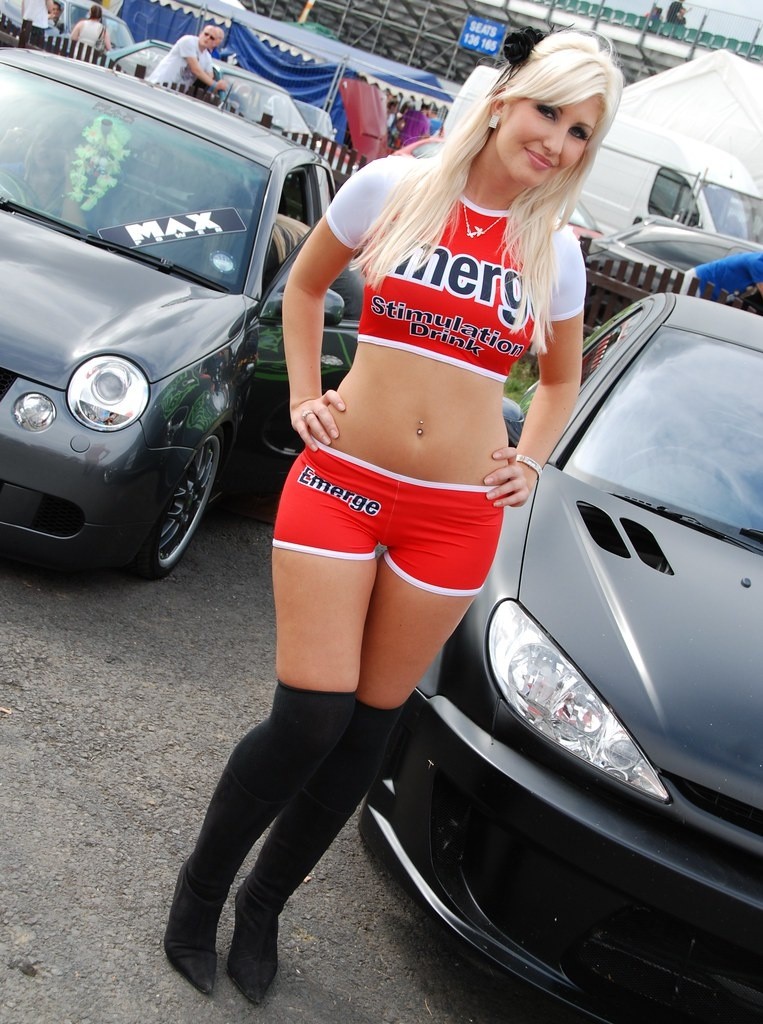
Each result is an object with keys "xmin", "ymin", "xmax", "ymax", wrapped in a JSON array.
[{"xmin": 582, "ymin": 213, "xmax": 763, "ymax": 343}]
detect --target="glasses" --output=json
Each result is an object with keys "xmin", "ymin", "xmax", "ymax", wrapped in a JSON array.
[{"xmin": 203, "ymin": 31, "xmax": 216, "ymax": 41}]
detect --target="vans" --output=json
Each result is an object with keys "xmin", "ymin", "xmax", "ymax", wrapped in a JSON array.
[{"xmin": 0, "ymin": 1, "xmax": 136, "ymax": 66}]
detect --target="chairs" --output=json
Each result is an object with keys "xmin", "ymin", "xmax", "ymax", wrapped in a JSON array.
[{"xmin": 110, "ymin": 174, "xmax": 189, "ymax": 229}]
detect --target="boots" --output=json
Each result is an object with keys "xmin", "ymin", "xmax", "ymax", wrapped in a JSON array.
[
  {"xmin": 226, "ymin": 789, "xmax": 356, "ymax": 1003},
  {"xmin": 164, "ymin": 763, "xmax": 292, "ymax": 994}
]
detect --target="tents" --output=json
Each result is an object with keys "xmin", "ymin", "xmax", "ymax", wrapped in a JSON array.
[
  {"xmin": 116, "ymin": 0, "xmax": 453, "ymax": 146},
  {"xmin": 618, "ymin": 48, "xmax": 763, "ymax": 199}
]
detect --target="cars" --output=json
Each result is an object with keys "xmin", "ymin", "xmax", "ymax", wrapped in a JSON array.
[
  {"xmin": 99, "ymin": 38, "xmax": 356, "ymax": 176},
  {"xmin": 0, "ymin": 40, "xmax": 366, "ymax": 599},
  {"xmin": 261, "ymin": 93, "xmax": 359, "ymax": 177},
  {"xmin": 358, "ymin": 286, "xmax": 763, "ymax": 1024}
]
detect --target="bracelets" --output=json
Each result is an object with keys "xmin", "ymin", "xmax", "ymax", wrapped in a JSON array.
[
  {"xmin": 517, "ymin": 454, "xmax": 542, "ymax": 479},
  {"xmin": 208, "ymin": 81, "xmax": 217, "ymax": 92}
]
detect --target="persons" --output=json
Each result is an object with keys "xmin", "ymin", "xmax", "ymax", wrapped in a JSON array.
[
  {"xmin": 165, "ymin": 28, "xmax": 623, "ymax": 1005},
  {"xmin": 70, "ymin": 5, "xmax": 111, "ymax": 63},
  {"xmin": 679, "ymin": 252, "xmax": 763, "ymax": 301},
  {"xmin": 22, "ymin": 0, "xmax": 60, "ymax": 47},
  {"xmin": 146, "ymin": 24, "xmax": 226, "ymax": 93},
  {"xmin": 387, "ymin": 100, "xmax": 443, "ymax": 149}
]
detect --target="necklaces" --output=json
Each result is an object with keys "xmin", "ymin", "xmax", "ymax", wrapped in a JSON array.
[{"xmin": 462, "ymin": 190, "xmax": 508, "ymax": 238}]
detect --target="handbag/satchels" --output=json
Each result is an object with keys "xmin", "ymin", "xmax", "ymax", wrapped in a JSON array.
[{"xmin": 95, "ymin": 26, "xmax": 106, "ymax": 52}]
